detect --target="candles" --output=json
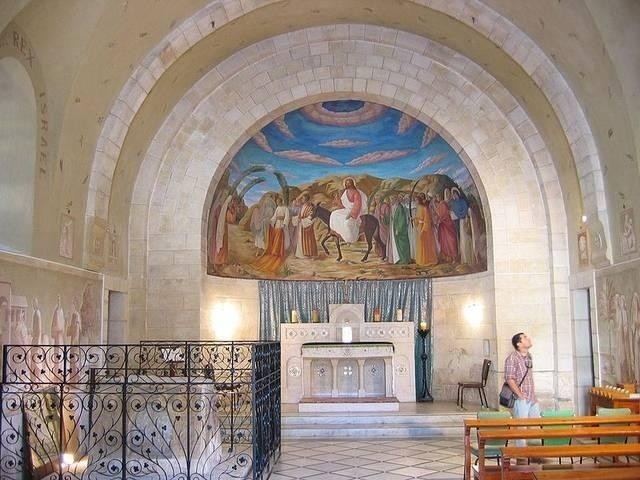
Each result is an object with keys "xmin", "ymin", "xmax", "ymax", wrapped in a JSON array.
[{"xmin": 420, "ymin": 322, "xmax": 427, "ymax": 329}]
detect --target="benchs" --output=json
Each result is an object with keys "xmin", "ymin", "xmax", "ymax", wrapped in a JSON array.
[{"xmin": 465, "ymin": 414, "xmax": 640, "ymax": 480}]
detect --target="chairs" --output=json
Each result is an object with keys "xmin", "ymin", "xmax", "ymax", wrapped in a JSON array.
[
  {"xmin": 469, "ymin": 407, "xmax": 632, "ymax": 464},
  {"xmin": 456, "ymin": 359, "xmax": 491, "ymax": 409}
]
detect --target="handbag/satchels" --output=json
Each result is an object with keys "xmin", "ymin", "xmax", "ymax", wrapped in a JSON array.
[{"xmin": 499, "ymin": 382, "xmax": 515, "ymax": 408}]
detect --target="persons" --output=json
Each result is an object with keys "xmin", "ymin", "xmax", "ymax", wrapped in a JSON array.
[
  {"xmin": 249, "ymin": 200, "xmax": 274, "ymax": 257},
  {"xmin": 287, "ymin": 199, "xmax": 302, "ymax": 259},
  {"xmin": 608, "ymin": 288, "xmax": 639, "ymax": 387},
  {"xmin": 578, "ymin": 237, "xmax": 589, "ymax": 265},
  {"xmin": 0, "ymin": 281, "xmax": 100, "ymax": 392},
  {"xmin": 504, "ymin": 332, "xmax": 553, "ymax": 466},
  {"xmin": 326, "ymin": 176, "xmax": 368, "ymax": 246},
  {"xmin": 372, "ymin": 185, "xmax": 473, "ymax": 267},
  {"xmin": 620, "ymin": 213, "xmax": 636, "ymax": 253},
  {"xmin": 263, "ymin": 197, "xmax": 290, "ymax": 257},
  {"xmin": 298, "ymin": 193, "xmax": 318, "ymax": 259}
]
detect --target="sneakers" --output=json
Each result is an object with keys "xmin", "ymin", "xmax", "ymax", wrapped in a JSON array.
[
  {"xmin": 531, "ymin": 457, "xmax": 553, "ymax": 464},
  {"xmin": 517, "ymin": 458, "xmax": 528, "ymax": 465}
]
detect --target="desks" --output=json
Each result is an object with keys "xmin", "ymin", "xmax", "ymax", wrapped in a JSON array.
[{"xmin": 589, "ymin": 392, "xmax": 639, "ymax": 441}]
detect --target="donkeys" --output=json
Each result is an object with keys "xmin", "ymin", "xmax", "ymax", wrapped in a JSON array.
[{"xmin": 307, "ymin": 200, "xmax": 387, "ymax": 263}]
